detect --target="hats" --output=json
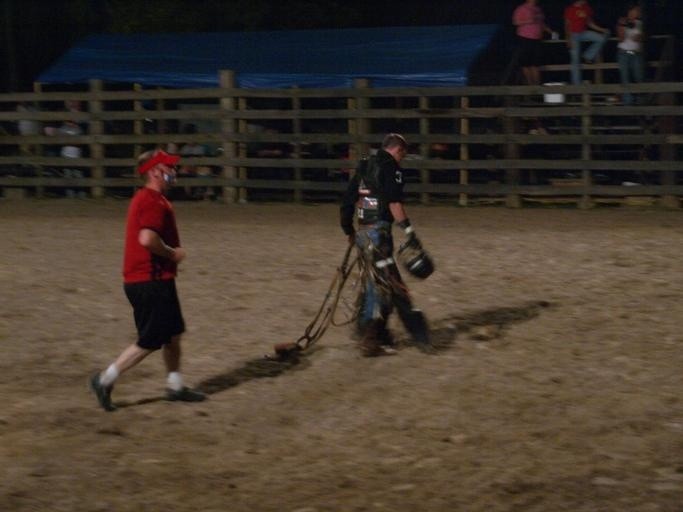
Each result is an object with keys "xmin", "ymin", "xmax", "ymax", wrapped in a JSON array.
[{"xmin": 137, "ymin": 150, "xmax": 181, "ymax": 175}]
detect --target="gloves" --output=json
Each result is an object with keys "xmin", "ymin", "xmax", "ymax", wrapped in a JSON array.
[{"xmin": 396, "ymin": 217, "xmax": 417, "ymax": 244}]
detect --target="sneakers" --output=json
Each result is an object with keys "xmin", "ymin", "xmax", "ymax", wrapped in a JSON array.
[
  {"xmin": 349, "ymin": 334, "xmax": 399, "ymax": 359},
  {"xmin": 161, "ymin": 387, "xmax": 205, "ymax": 401},
  {"xmin": 89, "ymin": 370, "xmax": 117, "ymax": 411}
]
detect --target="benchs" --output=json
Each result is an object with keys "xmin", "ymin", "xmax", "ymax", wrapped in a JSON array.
[{"xmin": 542, "ymin": 36, "xmax": 683, "ymax": 154}]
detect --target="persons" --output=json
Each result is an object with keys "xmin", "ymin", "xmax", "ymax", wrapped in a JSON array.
[
  {"xmin": 615, "ymin": 1, "xmax": 649, "ymax": 107},
  {"xmin": 562, "ymin": 1, "xmax": 610, "ymax": 104},
  {"xmin": 512, "ymin": 0, "xmax": 559, "ymax": 105},
  {"xmin": 86, "ymin": 148, "xmax": 207, "ymax": 414},
  {"xmin": 1, "ymin": 76, "xmax": 657, "ymax": 212},
  {"xmin": 337, "ymin": 133, "xmax": 437, "ymax": 356}
]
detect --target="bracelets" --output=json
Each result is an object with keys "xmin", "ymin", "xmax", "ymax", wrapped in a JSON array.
[
  {"xmin": 165, "ymin": 246, "xmax": 176, "ymax": 259},
  {"xmin": 399, "ymin": 219, "xmax": 410, "ymax": 229}
]
